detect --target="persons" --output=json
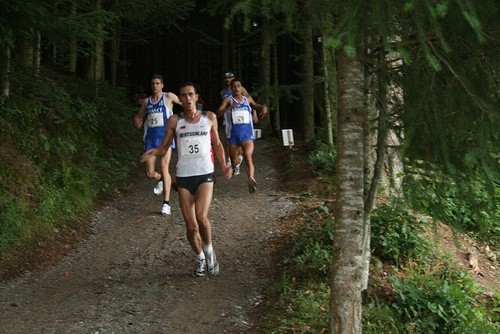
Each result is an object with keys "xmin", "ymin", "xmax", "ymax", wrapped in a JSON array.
[
  {"xmin": 216, "ymin": 80, "xmax": 268, "ymax": 194},
  {"xmin": 215, "ymin": 66, "xmax": 257, "ymax": 166},
  {"xmin": 140, "ymin": 82, "xmax": 231, "ymax": 277},
  {"xmin": 134, "ymin": 74, "xmax": 180, "ymax": 217}
]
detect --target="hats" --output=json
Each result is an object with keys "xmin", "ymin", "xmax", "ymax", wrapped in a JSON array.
[{"xmin": 225, "ymin": 72, "xmax": 234, "ymax": 77}]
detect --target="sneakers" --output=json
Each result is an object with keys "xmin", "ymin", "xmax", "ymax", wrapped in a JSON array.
[
  {"xmin": 153, "ymin": 180, "xmax": 163, "ymax": 196},
  {"xmin": 248, "ymin": 177, "xmax": 257, "ymax": 194},
  {"xmin": 193, "ymin": 257, "xmax": 206, "ymax": 277},
  {"xmin": 162, "ymin": 204, "xmax": 171, "ymax": 216},
  {"xmin": 227, "ymin": 154, "xmax": 243, "ymax": 176},
  {"xmin": 205, "ymin": 251, "xmax": 219, "ymax": 276}
]
